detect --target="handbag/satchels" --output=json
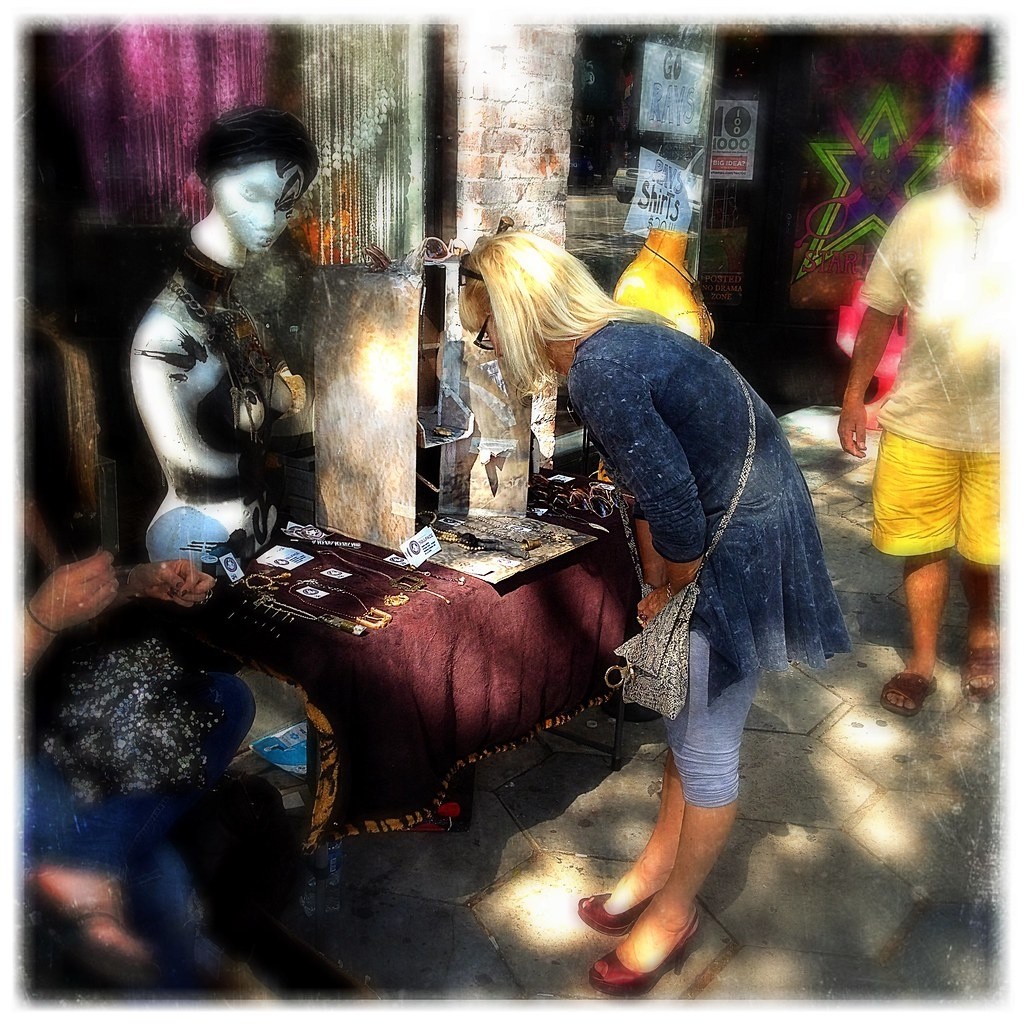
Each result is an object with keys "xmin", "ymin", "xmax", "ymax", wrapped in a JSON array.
[{"xmin": 603, "ymin": 582, "xmax": 700, "ymax": 721}]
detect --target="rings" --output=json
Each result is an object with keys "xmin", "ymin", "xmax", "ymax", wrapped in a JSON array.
[{"xmin": 638, "ymin": 614, "xmax": 648, "ymax": 620}]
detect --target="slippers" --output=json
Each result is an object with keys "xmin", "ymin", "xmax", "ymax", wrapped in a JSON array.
[
  {"xmin": 961, "ymin": 644, "xmax": 1001, "ymax": 703},
  {"xmin": 881, "ymin": 670, "xmax": 938, "ymax": 717}
]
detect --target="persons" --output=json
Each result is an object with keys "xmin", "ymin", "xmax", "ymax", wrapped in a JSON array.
[
  {"xmin": 128, "ymin": 106, "xmax": 319, "ymax": 600},
  {"xmin": 838, "ymin": 75, "xmax": 998, "ymax": 719},
  {"xmin": 605, "ymin": 222, "xmax": 719, "ymax": 347},
  {"xmin": 455, "ymin": 231, "xmax": 855, "ymax": 994},
  {"xmin": 22, "ymin": 325, "xmax": 292, "ymax": 1002}
]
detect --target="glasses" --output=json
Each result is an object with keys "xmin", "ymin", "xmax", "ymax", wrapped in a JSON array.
[{"xmin": 471, "ymin": 315, "xmax": 495, "ymax": 350}]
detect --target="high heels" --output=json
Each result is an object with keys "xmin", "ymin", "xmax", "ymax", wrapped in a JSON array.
[{"xmin": 577, "ymin": 893, "xmax": 700, "ymax": 996}]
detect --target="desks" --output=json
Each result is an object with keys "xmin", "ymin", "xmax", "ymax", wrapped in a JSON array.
[{"xmin": 129, "ymin": 468, "xmax": 738, "ymax": 956}]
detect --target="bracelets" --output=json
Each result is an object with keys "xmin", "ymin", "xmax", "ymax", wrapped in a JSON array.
[
  {"xmin": 666, "ymin": 582, "xmax": 673, "ymax": 599},
  {"xmin": 527, "ymin": 467, "xmax": 637, "ymax": 528},
  {"xmin": 116, "ymin": 558, "xmax": 145, "ymax": 602},
  {"xmin": 25, "ymin": 599, "xmax": 61, "ymax": 637}
]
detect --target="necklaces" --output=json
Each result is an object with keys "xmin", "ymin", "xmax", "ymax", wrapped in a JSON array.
[
  {"xmin": 165, "ymin": 272, "xmax": 280, "ymax": 451},
  {"xmin": 642, "ymin": 241, "xmax": 705, "ymax": 306},
  {"xmin": 202, "ymin": 503, "xmax": 571, "ymax": 637}
]
